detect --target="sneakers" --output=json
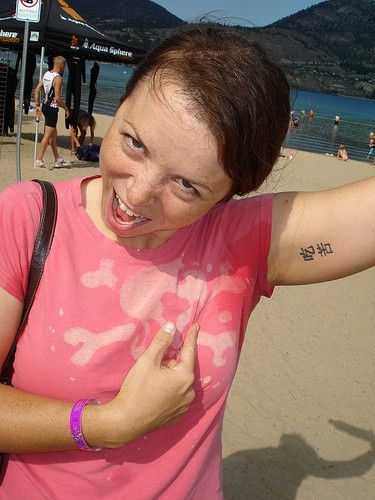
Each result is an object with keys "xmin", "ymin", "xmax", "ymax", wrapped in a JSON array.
[
  {"xmin": 35, "ymin": 160, "xmax": 53, "ymax": 170},
  {"xmin": 54, "ymin": 158, "xmax": 71, "ymax": 168}
]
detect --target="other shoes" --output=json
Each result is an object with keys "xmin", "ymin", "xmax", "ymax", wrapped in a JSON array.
[{"xmin": 71, "ymin": 150, "xmax": 75, "ymax": 156}]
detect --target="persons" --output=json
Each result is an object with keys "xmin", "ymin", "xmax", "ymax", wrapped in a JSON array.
[
  {"xmin": 33, "ymin": 56, "xmax": 71, "ymax": 170},
  {"xmin": 289, "ymin": 108, "xmax": 340, "ymax": 128},
  {"xmin": 367, "ymin": 132, "xmax": 375, "ymax": 159},
  {"xmin": 68, "ymin": 109, "xmax": 96, "ymax": 155},
  {"xmin": 337, "ymin": 144, "xmax": 348, "ymax": 161},
  {"xmin": 0, "ymin": 13, "xmax": 375, "ymax": 499}
]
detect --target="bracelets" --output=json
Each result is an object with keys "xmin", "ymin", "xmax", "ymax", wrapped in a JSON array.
[{"xmin": 69, "ymin": 398, "xmax": 104, "ymax": 451}]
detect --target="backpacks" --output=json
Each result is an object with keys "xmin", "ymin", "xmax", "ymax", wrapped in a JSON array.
[{"xmin": 75, "ymin": 144, "xmax": 100, "ymax": 162}]
